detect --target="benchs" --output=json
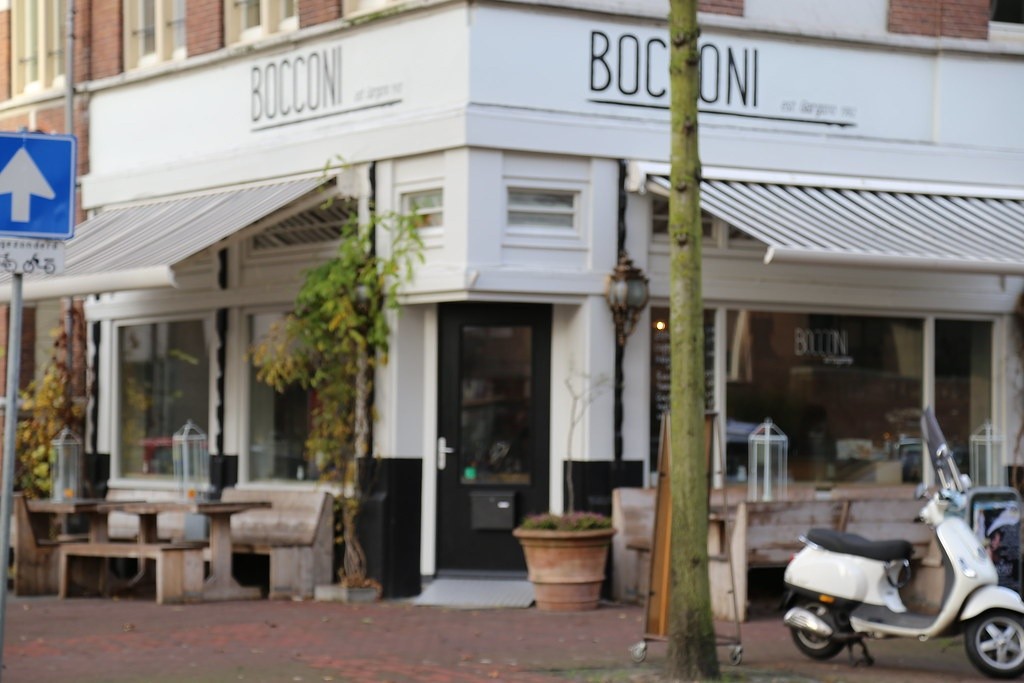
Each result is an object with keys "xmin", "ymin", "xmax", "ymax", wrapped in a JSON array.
[
  {"xmin": 103, "ymin": 488, "xmax": 334, "ymax": 600},
  {"xmin": 57, "ymin": 543, "xmax": 210, "ymax": 604}
]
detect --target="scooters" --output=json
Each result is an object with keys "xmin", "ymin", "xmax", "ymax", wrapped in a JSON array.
[{"xmin": 779, "ymin": 482, "xmax": 1024, "ymax": 679}]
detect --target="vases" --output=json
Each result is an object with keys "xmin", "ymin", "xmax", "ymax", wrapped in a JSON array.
[{"xmin": 513, "ymin": 527, "xmax": 618, "ymax": 611}]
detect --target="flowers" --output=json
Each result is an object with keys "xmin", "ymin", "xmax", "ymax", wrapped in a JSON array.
[{"xmin": 522, "ymin": 511, "xmax": 612, "ymax": 531}]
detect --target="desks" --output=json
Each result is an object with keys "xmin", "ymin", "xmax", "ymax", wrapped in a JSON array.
[
  {"xmin": 28, "ymin": 496, "xmax": 105, "ymax": 543},
  {"xmin": 96, "ymin": 499, "xmax": 271, "ymax": 599}
]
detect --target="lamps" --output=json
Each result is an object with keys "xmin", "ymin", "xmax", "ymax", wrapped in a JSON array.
[{"xmin": 606, "ymin": 249, "xmax": 651, "ymax": 347}]
